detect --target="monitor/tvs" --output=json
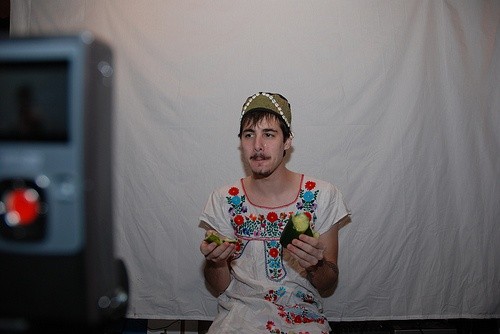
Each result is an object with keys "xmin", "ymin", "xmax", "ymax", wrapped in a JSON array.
[{"xmin": 0, "ymin": 57, "xmax": 71, "ymax": 144}]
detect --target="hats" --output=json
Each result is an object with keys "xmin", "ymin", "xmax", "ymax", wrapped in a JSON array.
[{"xmin": 239, "ymin": 92, "xmax": 292, "ymax": 129}]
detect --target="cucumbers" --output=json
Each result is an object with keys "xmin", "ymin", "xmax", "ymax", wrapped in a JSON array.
[{"xmin": 279, "ymin": 214, "xmax": 319, "ymax": 250}]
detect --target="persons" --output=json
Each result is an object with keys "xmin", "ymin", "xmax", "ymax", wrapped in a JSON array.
[{"xmin": 199, "ymin": 92, "xmax": 352, "ymax": 334}]
